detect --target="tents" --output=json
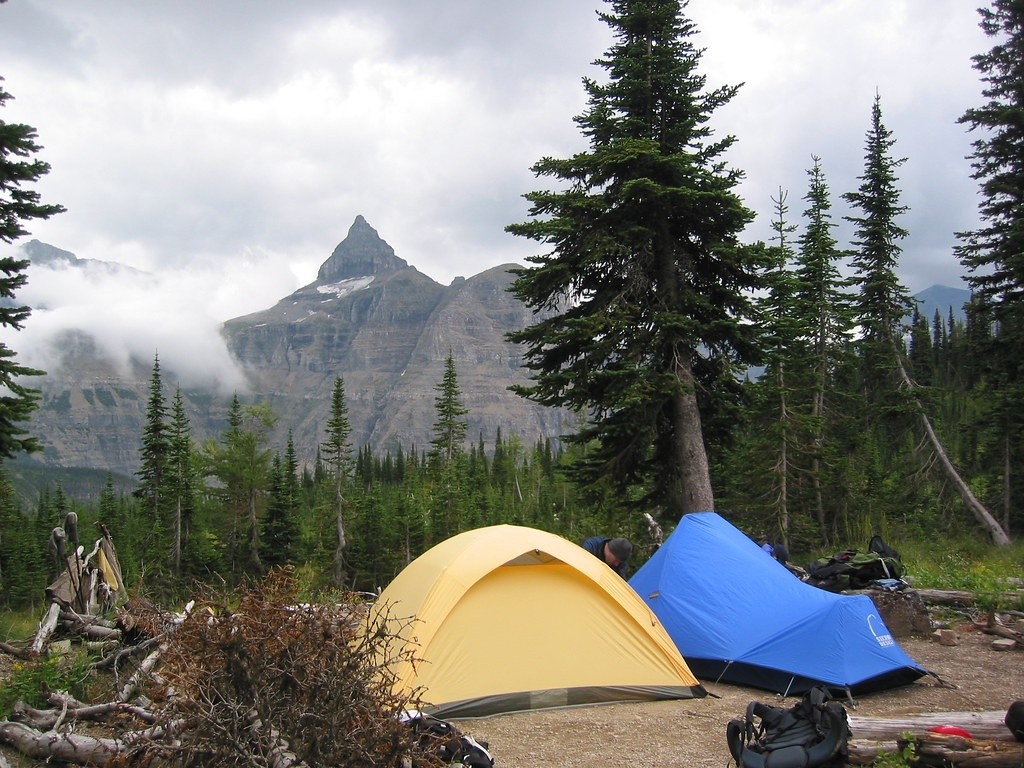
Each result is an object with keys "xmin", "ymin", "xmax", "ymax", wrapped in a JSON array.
[{"xmin": 339, "ymin": 511, "xmax": 959, "ymax": 723}]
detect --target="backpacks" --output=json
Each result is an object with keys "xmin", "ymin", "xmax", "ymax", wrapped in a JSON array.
[
  {"xmin": 869, "ymin": 535, "xmax": 901, "ymax": 563},
  {"xmin": 727, "ymin": 687, "xmax": 852, "ymax": 768}
]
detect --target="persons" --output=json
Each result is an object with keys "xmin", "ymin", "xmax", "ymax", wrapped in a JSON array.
[{"xmin": 582, "ymin": 537, "xmax": 632, "ymax": 571}]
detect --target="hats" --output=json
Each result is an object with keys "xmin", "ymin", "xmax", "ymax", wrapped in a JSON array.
[{"xmin": 608, "ymin": 538, "xmax": 632, "ymax": 562}]
